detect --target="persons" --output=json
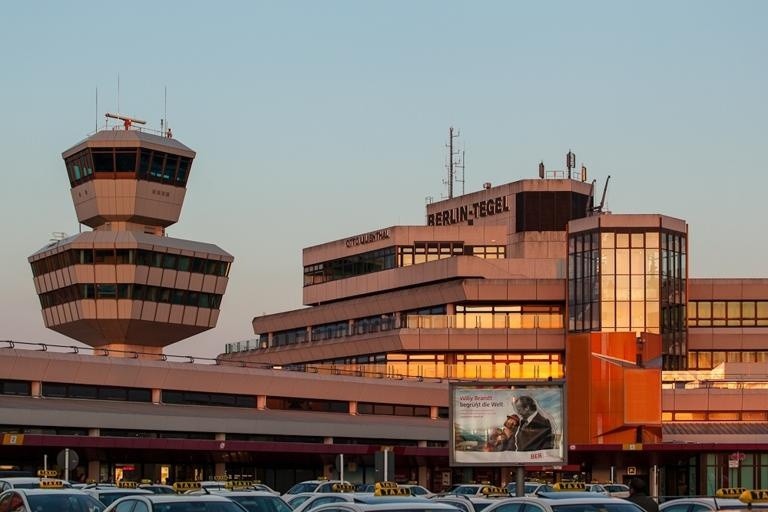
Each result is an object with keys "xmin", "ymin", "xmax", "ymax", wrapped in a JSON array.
[{"xmin": 487, "ymin": 396, "xmax": 555, "ymax": 452}]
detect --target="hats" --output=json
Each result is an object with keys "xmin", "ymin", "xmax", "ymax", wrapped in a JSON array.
[{"xmin": 507, "ymin": 414, "xmax": 519, "ymax": 424}]
